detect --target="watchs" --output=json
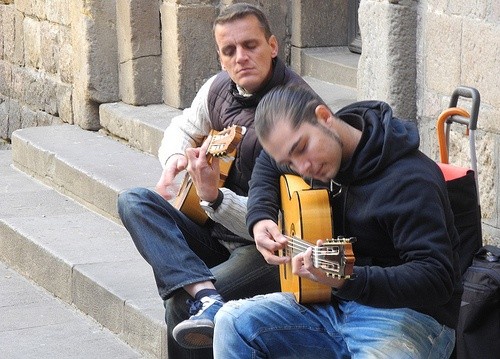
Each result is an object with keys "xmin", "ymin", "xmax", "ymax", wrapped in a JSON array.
[{"xmin": 200, "ymin": 190, "xmax": 223, "ymax": 213}]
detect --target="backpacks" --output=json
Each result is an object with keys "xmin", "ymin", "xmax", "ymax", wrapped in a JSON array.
[{"xmin": 457, "ymin": 246, "xmax": 500, "ymax": 359}]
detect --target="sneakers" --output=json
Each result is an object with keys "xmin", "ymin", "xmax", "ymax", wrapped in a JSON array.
[{"xmin": 173, "ymin": 293, "xmax": 224, "ymax": 348}]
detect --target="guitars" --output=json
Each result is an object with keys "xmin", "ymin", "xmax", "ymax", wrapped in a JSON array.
[
  {"xmin": 173, "ymin": 124, "xmax": 242, "ymax": 225},
  {"xmin": 275, "ymin": 173, "xmax": 359, "ymax": 305}
]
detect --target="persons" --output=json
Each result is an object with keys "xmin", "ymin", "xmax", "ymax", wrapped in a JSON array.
[
  {"xmin": 119, "ymin": 3, "xmax": 322, "ymax": 359},
  {"xmin": 213, "ymin": 81, "xmax": 463, "ymax": 359}
]
